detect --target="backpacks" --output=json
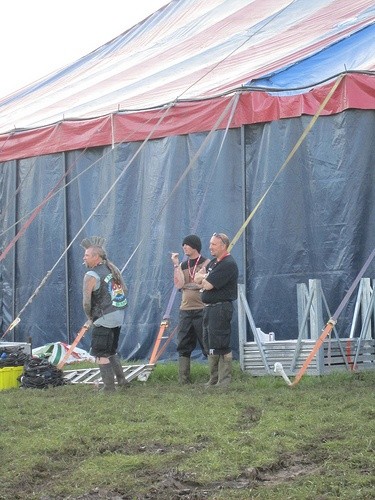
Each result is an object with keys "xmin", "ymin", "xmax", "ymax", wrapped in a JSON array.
[{"xmin": 0, "ymin": 350, "xmax": 66, "ymax": 389}]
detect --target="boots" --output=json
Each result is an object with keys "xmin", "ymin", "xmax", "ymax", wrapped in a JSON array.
[
  {"xmin": 176, "ymin": 356, "xmax": 191, "ymax": 385},
  {"xmin": 204, "ymin": 354, "xmax": 233, "ymax": 387},
  {"xmin": 97, "ymin": 354, "xmax": 130, "ymax": 393}
]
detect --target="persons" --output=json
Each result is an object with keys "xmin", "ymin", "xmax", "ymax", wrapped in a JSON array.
[
  {"xmin": 194, "ymin": 233, "xmax": 238, "ymax": 387},
  {"xmin": 81, "ymin": 236, "xmax": 130, "ymax": 391},
  {"xmin": 171, "ymin": 235, "xmax": 211, "ymax": 385}
]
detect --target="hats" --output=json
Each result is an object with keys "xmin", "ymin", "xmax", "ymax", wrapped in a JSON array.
[{"xmin": 181, "ymin": 235, "xmax": 201, "ymax": 250}]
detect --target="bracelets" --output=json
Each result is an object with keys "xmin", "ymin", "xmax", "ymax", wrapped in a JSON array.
[{"xmin": 174, "ymin": 265, "xmax": 178, "ymax": 267}]
